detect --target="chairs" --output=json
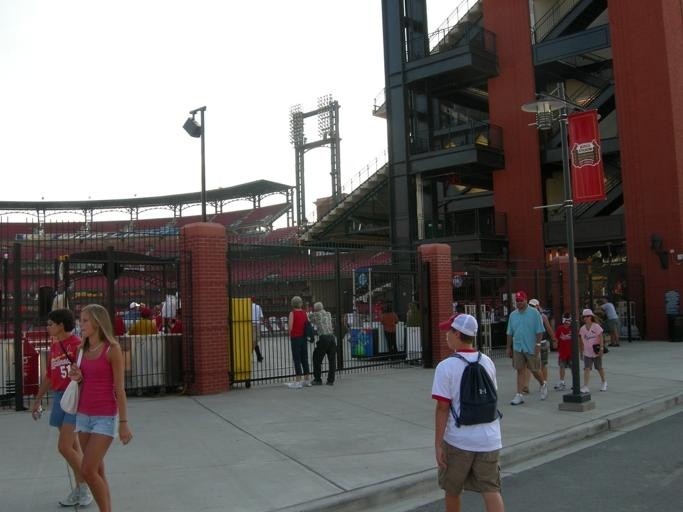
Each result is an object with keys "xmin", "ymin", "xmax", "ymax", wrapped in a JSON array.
[
  {"xmin": 2, "ymin": 218, "xmax": 179, "ymax": 334},
  {"xmin": 183, "ymin": 203, "xmax": 393, "ymax": 287}
]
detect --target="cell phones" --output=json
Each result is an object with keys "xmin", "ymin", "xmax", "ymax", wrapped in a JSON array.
[{"xmin": 33, "ymin": 404, "xmax": 42, "ymax": 418}]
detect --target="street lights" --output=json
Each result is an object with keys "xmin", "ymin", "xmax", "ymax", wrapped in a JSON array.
[
  {"xmin": 522, "ymin": 76, "xmax": 595, "ymax": 414},
  {"xmin": 181, "ymin": 105, "xmax": 211, "ymax": 221}
]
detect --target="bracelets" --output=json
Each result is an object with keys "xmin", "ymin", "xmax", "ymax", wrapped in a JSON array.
[{"xmin": 119, "ymin": 420, "xmax": 128, "ymax": 423}]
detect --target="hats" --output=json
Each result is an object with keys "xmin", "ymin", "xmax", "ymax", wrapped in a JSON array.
[
  {"xmin": 515, "ymin": 291, "xmax": 527, "ymax": 300},
  {"xmin": 438, "ymin": 313, "xmax": 480, "ymax": 337},
  {"xmin": 528, "ymin": 298, "xmax": 539, "ymax": 306},
  {"xmin": 582, "ymin": 309, "xmax": 596, "ymax": 316},
  {"xmin": 129, "ymin": 301, "xmax": 140, "ymax": 309}
]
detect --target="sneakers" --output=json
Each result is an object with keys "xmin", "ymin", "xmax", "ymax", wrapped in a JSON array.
[
  {"xmin": 580, "ymin": 386, "xmax": 590, "ymax": 393},
  {"xmin": 539, "ymin": 382, "xmax": 549, "ymax": 401},
  {"xmin": 599, "ymin": 382, "xmax": 609, "ymax": 391},
  {"xmin": 301, "ymin": 379, "xmax": 313, "ymax": 388},
  {"xmin": 59, "ymin": 486, "xmax": 80, "ymax": 508},
  {"xmin": 607, "ymin": 343, "xmax": 620, "ymax": 347},
  {"xmin": 510, "ymin": 393, "xmax": 524, "ymax": 405},
  {"xmin": 554, "ymin": 382, "xmax": 568, "ymax": 391},
  {"xmin": 77, "ymin": 480, "xmax": 94, "ymax": 506},
  {"xmin": 325, "ymin": 381, "xmax": 334, "ymax": 386},
  {"xmin": 287, "ymin": 379, "xmax": 303, "ymax": 389},
  {"xmin": 309, "ymin": 379, "xmax": 323, "ymax": 385}
]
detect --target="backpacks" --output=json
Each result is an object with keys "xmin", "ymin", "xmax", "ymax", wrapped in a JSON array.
[{"xmin": 448, "ymin": 353, "xmax": 499, "ymax": 425}]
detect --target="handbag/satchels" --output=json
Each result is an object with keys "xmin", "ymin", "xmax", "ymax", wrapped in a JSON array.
[
  {"xmin": 59, "ymin": 355, "xmax": 84, "ymax": 416},
  {"xmin": 304, "ymin": 318, "xmax": 316, "ymax": 339}
]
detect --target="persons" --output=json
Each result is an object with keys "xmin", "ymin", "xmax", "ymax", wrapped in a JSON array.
[
  {"xmin": 284, "ymin": 296, "xmax": 315, "ymax": 389},
  {"xmin": 431, "ymin": 311, "xmax": 506, "ymax": 512},
  {"xmin": 124, "ymin": 302, "xmax": 181, "ymax": 334},
  {"xmin": 438, "ymin": 304, "xmax": 466, "ymax": 332},
  {"xmin": 250, "ymin": 294, "xmax": 264, "ymax": 363},
  {"xmin": 51, "ymin": 285, "xmax": 74, "ymax": 312},
  {"xmin": 69, "ymin": 304, "xmax": 134, "ymax": 512},
  {"xmin": 504, "ymin": 290, "xmax": 620, "ymax": 407},
  {"xmin": 381, "ymin": 306, "xmax": 399, "ymax": 357},
  {"xmin": 308, "ymin": 303, "xmax": 337, "ymax": 386},
  {"xmin": 31, "ymin": 309, "xmax": 94, "ymax": 507}
]
